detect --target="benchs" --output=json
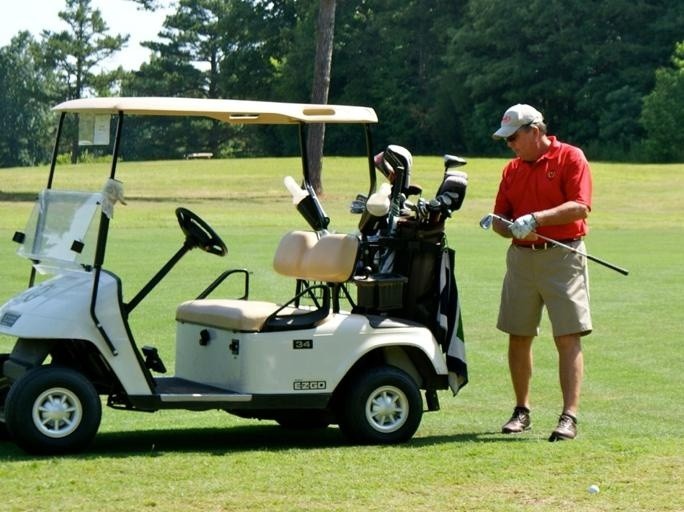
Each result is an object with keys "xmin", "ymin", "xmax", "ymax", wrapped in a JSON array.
[{"xmin": 175, "ymin": 229, "xmax": 360, "ymax": 334}]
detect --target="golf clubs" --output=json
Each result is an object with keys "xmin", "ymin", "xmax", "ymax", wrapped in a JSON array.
[
  {"xmin": 350, "ymin": 143, "xmax": 468, "ymax": 237},
  {"xmin": 480, "ymin": 214, "xmax": 629, "ymax": 276}
]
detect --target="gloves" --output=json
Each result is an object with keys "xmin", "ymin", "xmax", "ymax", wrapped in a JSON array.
[{"xmin": 507, "ymin": 214, "xmax": 539, "ymax": 239}]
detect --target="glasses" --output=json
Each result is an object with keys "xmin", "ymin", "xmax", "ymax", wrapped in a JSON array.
[{"xmin": 506, "ymin": 132, "xmax": 521, "ymax": 141}]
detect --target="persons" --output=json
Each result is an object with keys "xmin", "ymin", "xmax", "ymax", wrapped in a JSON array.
[{"xmin": 492, "ymin": 102, "xmax": 593, "ymax": 439}]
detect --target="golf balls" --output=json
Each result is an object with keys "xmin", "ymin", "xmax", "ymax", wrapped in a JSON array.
[{"xmin": 589, "ymin": 483, "xmax": 599, "ymax": 492}]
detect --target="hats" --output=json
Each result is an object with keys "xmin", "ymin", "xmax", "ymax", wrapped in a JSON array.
[{"xmin": 492, "ymin": 104, "xmax": 544, "ymax": 141}]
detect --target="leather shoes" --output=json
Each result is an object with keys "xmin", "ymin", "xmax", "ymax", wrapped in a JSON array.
[
  {"xmin": 552, "ymin": 413, "xmax": 577, "ymax": 440},
  {"xmin": 502, "ymin": 407, "xmax": 530, "ymax": 434}
]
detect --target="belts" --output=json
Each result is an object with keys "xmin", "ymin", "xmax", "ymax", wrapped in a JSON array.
[{"xmin": 520, "ymin": 238, "xmax": 582, "ymax": 249}]
